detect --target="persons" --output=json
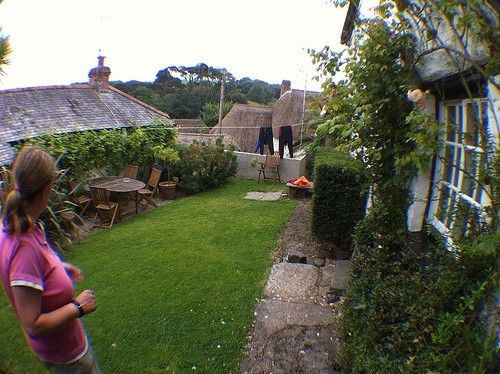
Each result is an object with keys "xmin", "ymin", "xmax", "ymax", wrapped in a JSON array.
[{"xmin": 0, "ymin": 145, "xmax": 100, "ymax": 374}]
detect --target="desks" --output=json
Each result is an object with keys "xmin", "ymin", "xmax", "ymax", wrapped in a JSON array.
[
  {"xmin": 88, "ymin": 176, "xmax": 146, "ymax": 220},
  {"xmin": 286, "ymin": 181, "xmax": 314, "ymax": 199}
]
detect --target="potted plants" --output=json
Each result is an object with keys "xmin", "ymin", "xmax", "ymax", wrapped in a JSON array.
[{"xmin": 154, "ymin": 146, "xmax": 181, "ymax": 200}]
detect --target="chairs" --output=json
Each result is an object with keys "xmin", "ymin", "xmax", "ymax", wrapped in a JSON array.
[
  {"xmin": 89, "ymin": 186, "xmax": 122, "ymax": 230},
  {"xmin": 257, "ymin": 151, "xmax": 281, "ymax": 185},
  {"xmin": 123, "ymin": 165, "xmax": 138, "ymax": 206},
  {"xmin": 138, "ymin": 167, "xmax": 162, "ymax": 211},
  {"xmin": 66, "ymin": 181, "xmax": 92, "ymax": 217}
]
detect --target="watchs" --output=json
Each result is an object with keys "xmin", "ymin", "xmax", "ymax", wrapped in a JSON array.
[{"xmin": 70, "ymin": 298, "xmax": 84, "ymax": 317}]
